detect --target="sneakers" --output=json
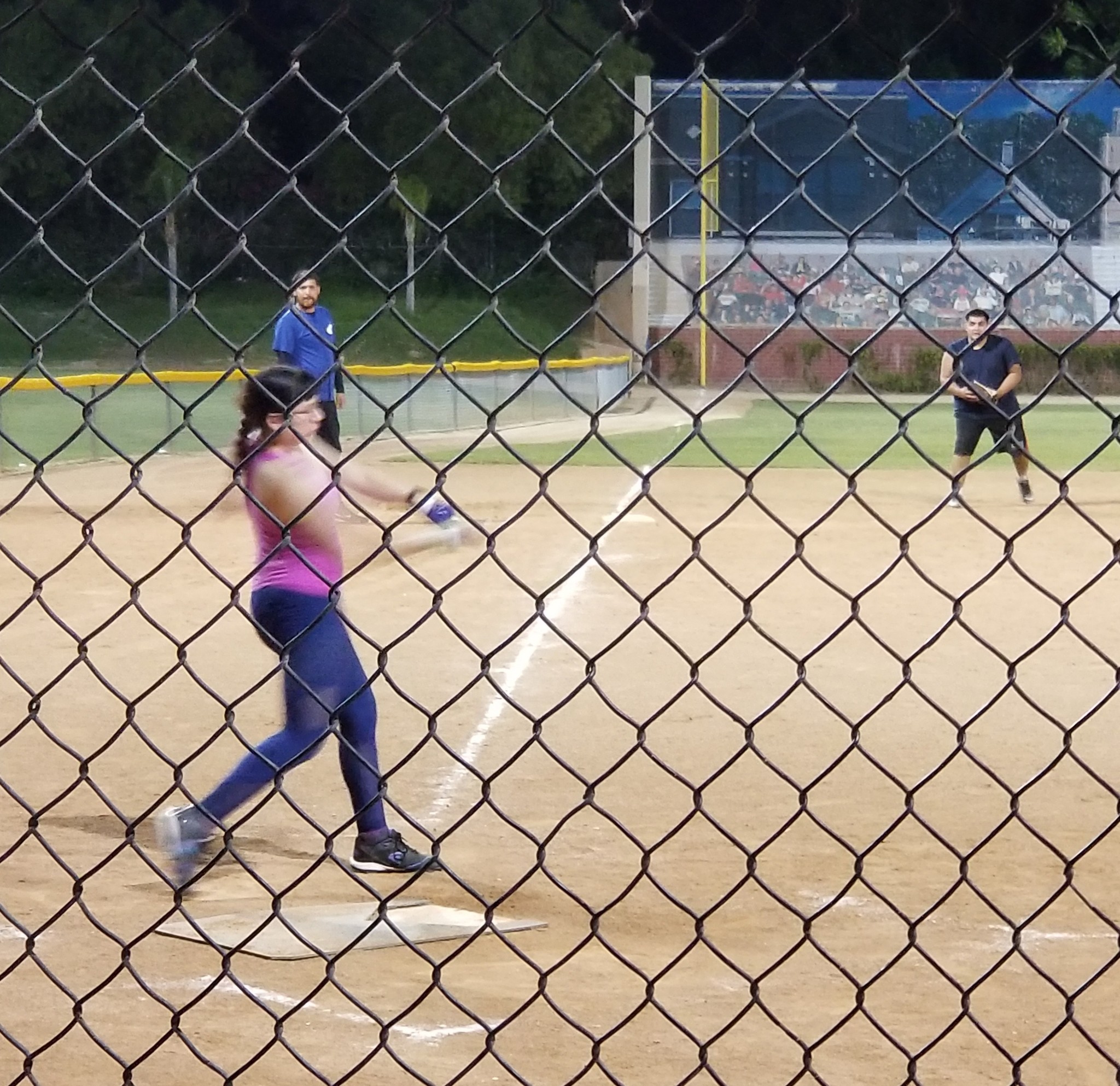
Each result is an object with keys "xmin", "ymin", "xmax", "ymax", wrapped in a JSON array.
[
  {"xmin": 350, "ymin": 828, "xmax": 441, "ymax": 871},
  {"xmin": 163, "ymin": 809, "xmax": 215, "ymax": 896}
]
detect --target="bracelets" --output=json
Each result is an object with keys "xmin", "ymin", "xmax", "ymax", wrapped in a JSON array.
[{"xmin": 406, "ymin": 490, "xmax": 419, "ymax": 504}]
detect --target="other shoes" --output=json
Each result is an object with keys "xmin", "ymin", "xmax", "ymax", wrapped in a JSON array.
[
  {"xmin": 1019, "ymin": 479, "xmax": 1034, "ymax": 501},
  {"xmin": 948, "ymin": 499, "xmax": 960, "ymax": 507}
]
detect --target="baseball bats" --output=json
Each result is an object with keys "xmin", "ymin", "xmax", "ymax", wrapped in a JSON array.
[{"xmin": 365, "ymin": 525, "xmax": 469, "ymax": 569}]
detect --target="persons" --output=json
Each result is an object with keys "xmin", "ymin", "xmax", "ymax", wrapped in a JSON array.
[
  {"xmin": 154, "ymin": 367, "xmax": 484, "ymax": 892},
  {"xmin": 272, "ymin": 267, "xmax": 347, "ymax": 453},
  {"xmin": 941, "ymin": 310, "xmax": 1034, "ymax": 506},
  {"xmin": 691, "ymin": 253, "xmax": 1095, "ymax": 329}
]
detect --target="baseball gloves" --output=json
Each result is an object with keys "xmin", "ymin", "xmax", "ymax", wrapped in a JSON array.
[{"xmin": 967, "ymin": 379, "xmax": 999, "ymax": 406}]
